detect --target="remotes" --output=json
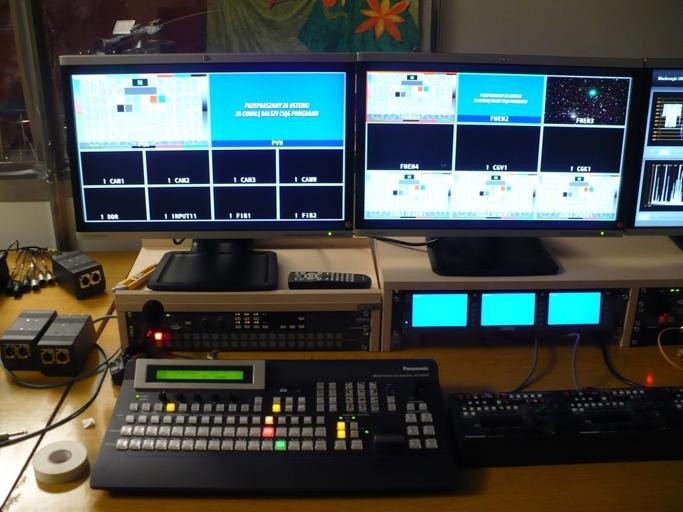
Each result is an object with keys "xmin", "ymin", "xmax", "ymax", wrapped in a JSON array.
[{"xmin": 288, "ymin": 272, "xmax": 371, "ymax": 289}]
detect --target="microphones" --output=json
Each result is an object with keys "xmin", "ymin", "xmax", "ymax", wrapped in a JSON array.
[{"xmin": 137, "ymin": 300, "xmax": 164, "ymax": 354}]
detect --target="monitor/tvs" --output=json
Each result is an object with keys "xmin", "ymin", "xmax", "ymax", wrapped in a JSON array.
[
  {"xmin": 354, "ymin": 52, "xmax": 643, "ymax": 276},
  {"xmin": 59, "ymin": 52, "xmax": 355, "ymax": 291},
  {"xmin": 625, "ymin": 57, "xmax": 683, "ymax": 252}
]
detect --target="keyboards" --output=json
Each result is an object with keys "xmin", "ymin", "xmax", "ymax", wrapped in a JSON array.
[{"xmin": 449, "ymin": 386, "xmax": 683, "ymax": 468}]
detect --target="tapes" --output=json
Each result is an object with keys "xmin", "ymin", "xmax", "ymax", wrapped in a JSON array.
[{"xmin": 31, "ymin": 438, "xmax": 90, "ymax": 485}]
